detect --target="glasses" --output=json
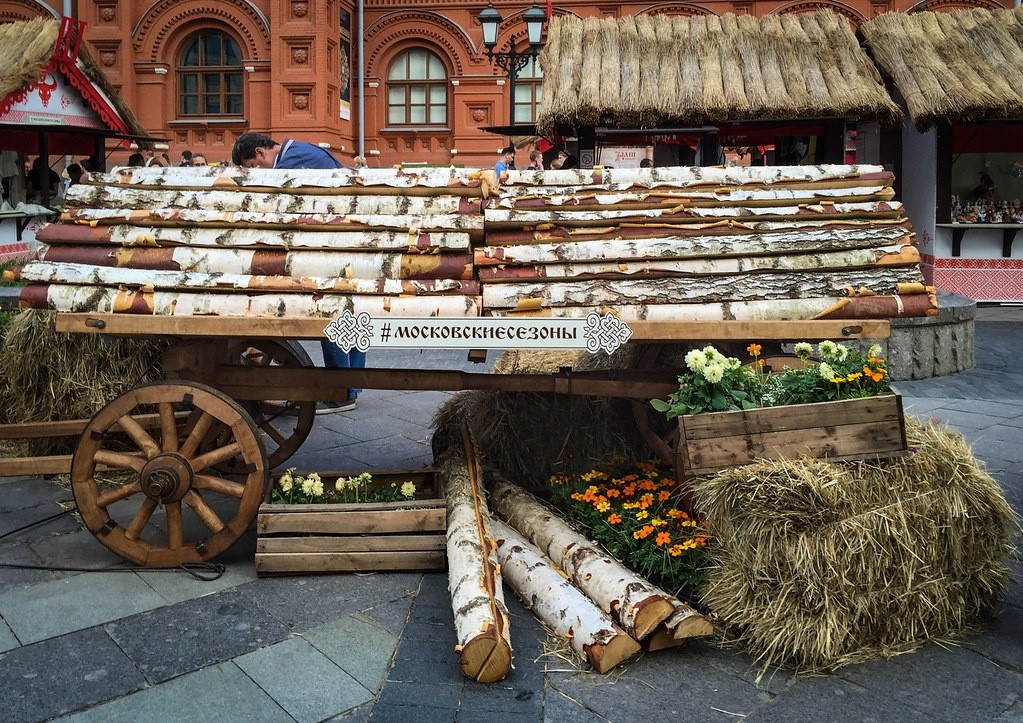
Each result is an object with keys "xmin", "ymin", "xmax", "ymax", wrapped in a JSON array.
[
  {"xmin": 194, "ymin": 163, "xmax": 205, "ymax": 166},
  {"xmin": 510, "ymin": 155, "xmax": 515, "ymax": 159}
]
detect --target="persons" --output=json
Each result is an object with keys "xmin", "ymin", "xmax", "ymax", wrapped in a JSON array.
[
  {"xmin": 66, "ymin": 163, "xmax": 83, "ymax": 187},
  {"xmin": 527, "ymin": 151, "xmax": 544, "ymax": 170},
  {"xmin": 191, "ymin": 153, "xmax": 208, "ymax": 166},
  {"xmin": 231, "ymin": 132, "xmax": 365, "ymax": 416},
  {"xmin": 951, "ymin": 194, "xmax": 1023, "ymax": 223},
  {"xmin": 128, "ymin": 154, "xmax": 145, "ymax": 166},
  {"xmin": 494, "ymin": 147, "xmax": 515, "ymax": 177},
  {"xmin": 550, "ymin": 150, "xmax": 570, "ymax": 170},
  {"xmin": 137, "ymin": 148, "xmax": 143, "ymax": 154},
  {"xmin": 640, "ymin": 158, "xmax": 652, "ymax": 168},
  {"xmin": 178, "ymin": 150, "xmax": 192, "ymax": 167},
  {"xmin": 145, "ymin": 151, "xmax": 154, "ymax": 167},
  {"xmin": 162, "ymin": 153, "xmax": 173, "ymax": 167},
  {"xmin": 217, "ymin": 159, "xmax": 228, "ymax": 167},
  {"xmin": 27, "ymin": 158, "xmax": 63, "ymax": 198}
]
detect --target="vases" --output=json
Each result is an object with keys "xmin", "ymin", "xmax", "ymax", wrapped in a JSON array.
[{"xmin": 677, "ymin": 383, "xmax": 907, "ymax": 477}]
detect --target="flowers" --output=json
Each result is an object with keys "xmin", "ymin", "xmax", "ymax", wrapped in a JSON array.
[
  {"xmin": 268, "ymin": 465, "xmax": 416, "ymax": 504},
  {"xmin": 649, "ymin": 338, "xmax": 892, "ymax": 422},
  {"xmin": 543, "ymin": 450, "xmax": 710, "ymax": 586}
]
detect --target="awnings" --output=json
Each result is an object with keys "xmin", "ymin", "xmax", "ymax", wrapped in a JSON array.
[{"xmin": 476, "ymin": 125, "xmax": 569, "ymax": 157}]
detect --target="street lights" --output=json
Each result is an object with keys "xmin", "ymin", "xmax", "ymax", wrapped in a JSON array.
[{"xmin": 477, "ymin": 1, "xmax": 548, "ymax": 170}]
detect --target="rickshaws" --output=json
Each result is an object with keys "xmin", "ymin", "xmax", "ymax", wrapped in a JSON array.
[{"xmin": 1, "ymin": 311, "xmax": 891, "ymax": 571}]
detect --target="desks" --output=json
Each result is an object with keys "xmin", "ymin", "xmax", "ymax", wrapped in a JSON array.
[{"xmin": 935, "ymin": 224, "xmax": 1023, "ymax": 257}]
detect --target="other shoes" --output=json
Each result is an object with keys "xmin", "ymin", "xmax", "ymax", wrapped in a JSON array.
[{"xmin": 314, "ymin": 399, "xmax": 357, "ymax": 414}]
